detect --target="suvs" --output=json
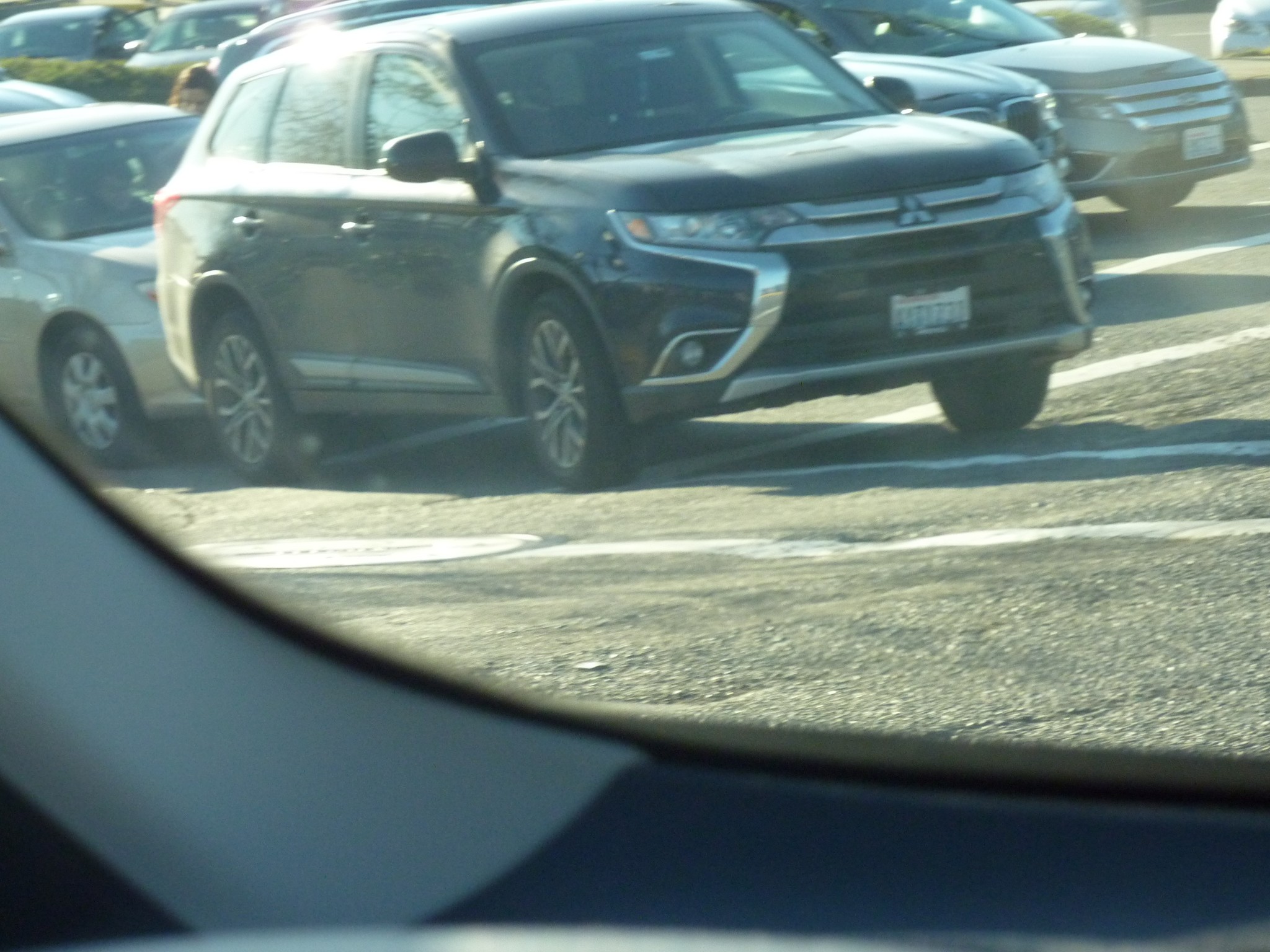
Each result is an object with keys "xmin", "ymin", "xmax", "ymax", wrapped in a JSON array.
[{"xmin": 148, "ymin": 0, "xmax": 1099, "ymax": 493}]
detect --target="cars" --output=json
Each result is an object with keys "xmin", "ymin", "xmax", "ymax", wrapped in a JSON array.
[
  {"xmin": 741, "ymin": 0, "xmax": 1270, "ymax": 218},
  {"xmin": 1, "ymin": 2, "xmax": 490, "ymax": 468}
]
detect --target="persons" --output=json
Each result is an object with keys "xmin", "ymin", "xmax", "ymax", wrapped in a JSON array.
[
  {"xmin": 168, "ymin": 64, "xmax": 216, "ymax": 118},
  {"xmin": 87, "ymin": 152, "xmax": 154, "ymax": 231}
]
